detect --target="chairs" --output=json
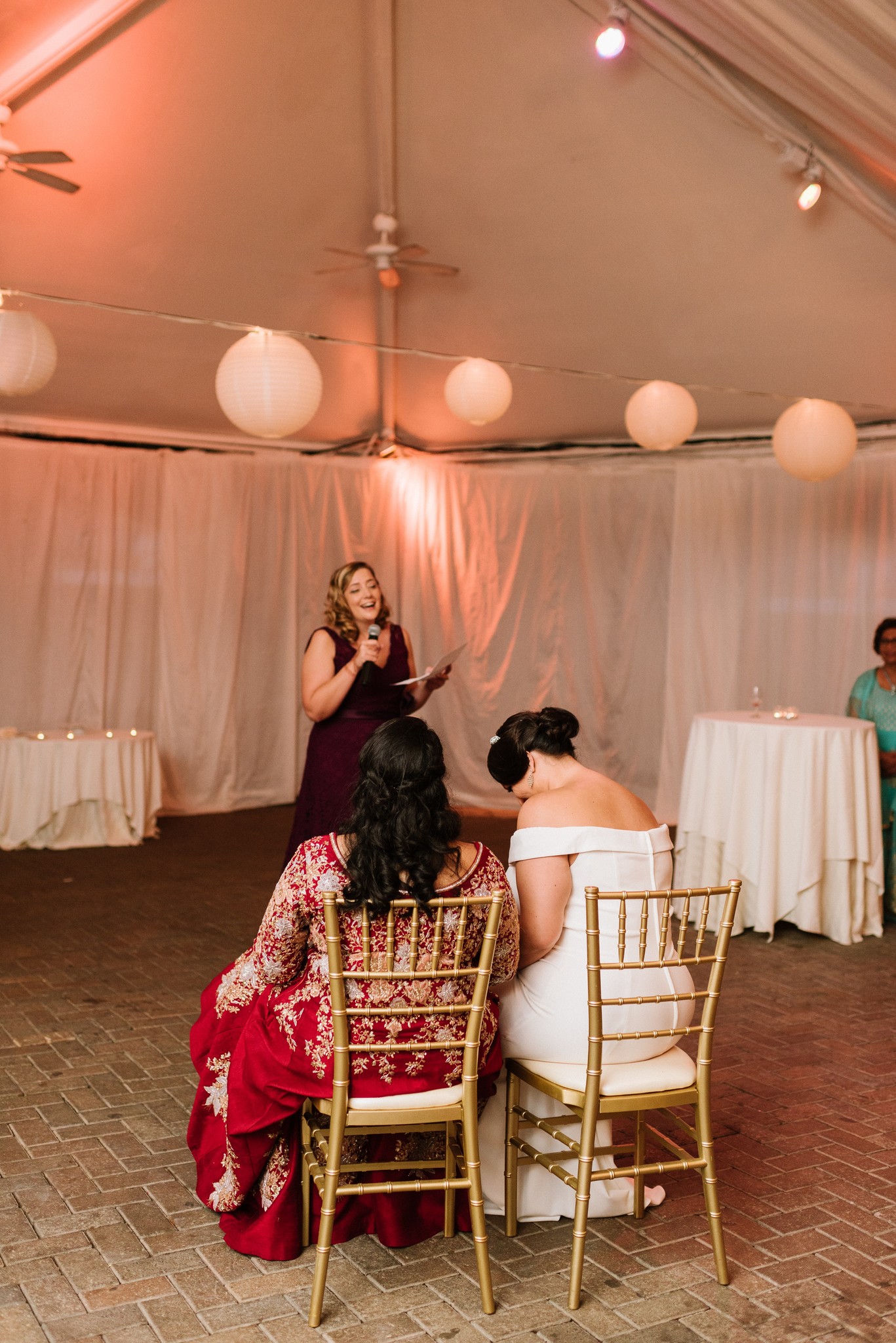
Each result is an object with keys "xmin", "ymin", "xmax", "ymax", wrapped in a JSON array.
[
  {"xmin": 501, "ymin": 876, "xmax": 738, "ymax": 1288},
  {"xmin": 297, "ymin": 891, "xmax": 508, "ymax": 1325}
]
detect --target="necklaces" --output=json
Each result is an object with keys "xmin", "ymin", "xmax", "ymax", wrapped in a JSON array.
[{"xmin": 883, "ymin": 665, "xmax": 896, "ymax": 697}]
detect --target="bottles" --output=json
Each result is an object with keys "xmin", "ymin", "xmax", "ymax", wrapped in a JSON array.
[{"xmin": 752, "ymin": 687, "xmax": 760, "ymax": 718}]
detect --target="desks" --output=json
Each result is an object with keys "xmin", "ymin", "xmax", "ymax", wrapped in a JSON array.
[
  {"xmin": 0, "ymin": 724, "xmax": 164, "ymax": 850},
  {"xmin": 678, "ymin": 707, "xmax": 883, "ymax": 937}
]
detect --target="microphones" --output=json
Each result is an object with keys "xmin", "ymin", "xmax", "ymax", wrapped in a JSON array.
[{"xmin": 363, "ymin": 623, "xmax": 381, "ymax": 689}]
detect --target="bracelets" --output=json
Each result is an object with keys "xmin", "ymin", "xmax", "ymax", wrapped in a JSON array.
[{"xmin": 343, "ymin": 664, "xmax": 358, "ymax": 678}]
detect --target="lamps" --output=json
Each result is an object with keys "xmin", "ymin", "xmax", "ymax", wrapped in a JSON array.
[
  {"xmin": 592, "ymin": 0, "xmax": 628, "ymax": 58},
  {"xmin": 791, "ymin": 150, "xmax": 824, "ymax": 210}
]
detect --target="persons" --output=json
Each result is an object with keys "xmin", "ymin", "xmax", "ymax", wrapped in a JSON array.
[
  {"xmin": 280, "ymin": 559, "xmax": 454, "ymax": 877},
  {"xmin": 475, "ymin": 706, "xmax": 699, "ymax": 1223},
  {"xmin": 845, "ymin": 617, "xmax": 896, "ymax": 927},
  {"xmin": 185, "ymin": 713, "xmax": 521, "ymax": 1264}
]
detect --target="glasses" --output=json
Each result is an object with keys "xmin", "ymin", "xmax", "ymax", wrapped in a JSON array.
[{"xmin": 878, "ymin": 637, "xmax": 896, "ymax": 648}]
